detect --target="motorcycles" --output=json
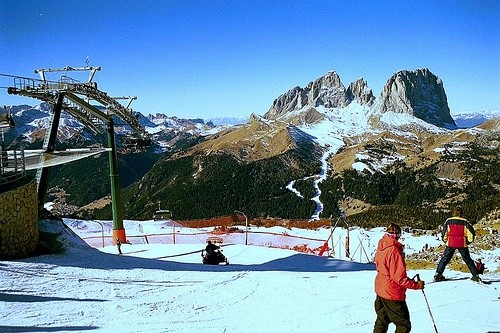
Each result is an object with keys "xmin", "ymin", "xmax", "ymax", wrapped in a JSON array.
[{"xmin": 202, "ymin": 247, "xmax": 229, "ymax": 265}]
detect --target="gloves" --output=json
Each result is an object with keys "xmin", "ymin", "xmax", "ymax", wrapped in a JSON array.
[{"xmin": 417, "ymin": 281, "xmax": 425, "ymax": 289}]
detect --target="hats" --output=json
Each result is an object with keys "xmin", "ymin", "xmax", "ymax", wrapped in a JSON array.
[
  {"xmin": 453, "ymin": 206, "xmax": 464, "ymax": 217},
  {"xmin": 387, "ymin": 224, "xmax": 401, "ymax": 234}
]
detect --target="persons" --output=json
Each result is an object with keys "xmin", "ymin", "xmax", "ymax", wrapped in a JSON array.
[
  {"xmin": 206, "ymin": 241, "xmax": 220, "ymax": 254},
  {"xmin": 433, "ymin": 208, "xmax": 482, "ymax": 282},
  {"xmin": 372, "ymin": 223, "xmax": 425, "ymax": 333}
]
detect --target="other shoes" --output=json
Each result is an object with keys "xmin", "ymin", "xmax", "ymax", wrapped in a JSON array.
[
  {"xmin": 471, "ymin": 275, "xmax": 481, "ymax": 282},
  {"xmin": 435, "ymin": 273, "xmax": 446, "ymax": 282}
]
